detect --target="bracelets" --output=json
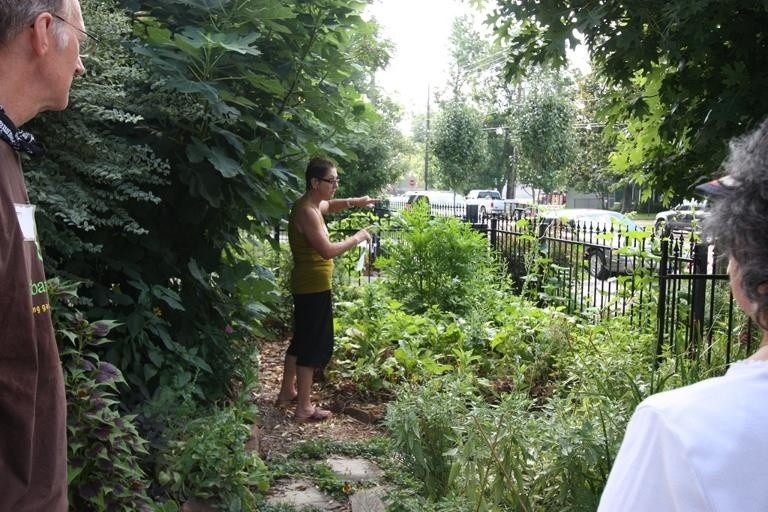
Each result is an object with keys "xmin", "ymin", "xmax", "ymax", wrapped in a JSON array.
[{"xmin": 346, "ymin": 197, "xmax": 355, "ymax": 209}]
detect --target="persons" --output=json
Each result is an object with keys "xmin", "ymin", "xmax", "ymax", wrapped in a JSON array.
[
  {"xmin": 274, "ymin": 157, "xmax": 379, "ymax": 422},
  {"xmin": 597, "ymin": 123, "xmax": 768, "ymax": 512},
  {"xmin": 0, "ymin": 0, "xmax": 99, "ymax": 512}
]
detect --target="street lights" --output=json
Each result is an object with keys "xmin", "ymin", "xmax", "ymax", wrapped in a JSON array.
[{"xmin": 495, "ymin": 125, "xmax": 517, "ymax": 199}]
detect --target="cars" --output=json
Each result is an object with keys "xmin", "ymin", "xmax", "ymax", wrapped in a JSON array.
[
  {"xmin": 519, "ymin": 207, "xmax": 689, "ymax": 281},
  {"xmin": 398, "ymin": 189, "xmax": 511, "ymax": 224},
  {"xmin": 653, "ymin": 202, "xmax": 713, "ymax": 238}
]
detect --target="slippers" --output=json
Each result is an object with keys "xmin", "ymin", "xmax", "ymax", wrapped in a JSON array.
[
  {"xmin": 294, "ymin": 408, "xmax": 331, "ymax": 423},
  {"xmin": 277, "ymin": 394, "xmax": 315, "ymax": 404}
]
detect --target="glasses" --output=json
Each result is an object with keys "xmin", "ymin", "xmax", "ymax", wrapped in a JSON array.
[
  {"xmin": 30, "ymin": 10, "xmax": 100, "ymax": 57},
  {"xmin": 321, "ymin": 178, "xmax": 340, "ymax": 184}
]
